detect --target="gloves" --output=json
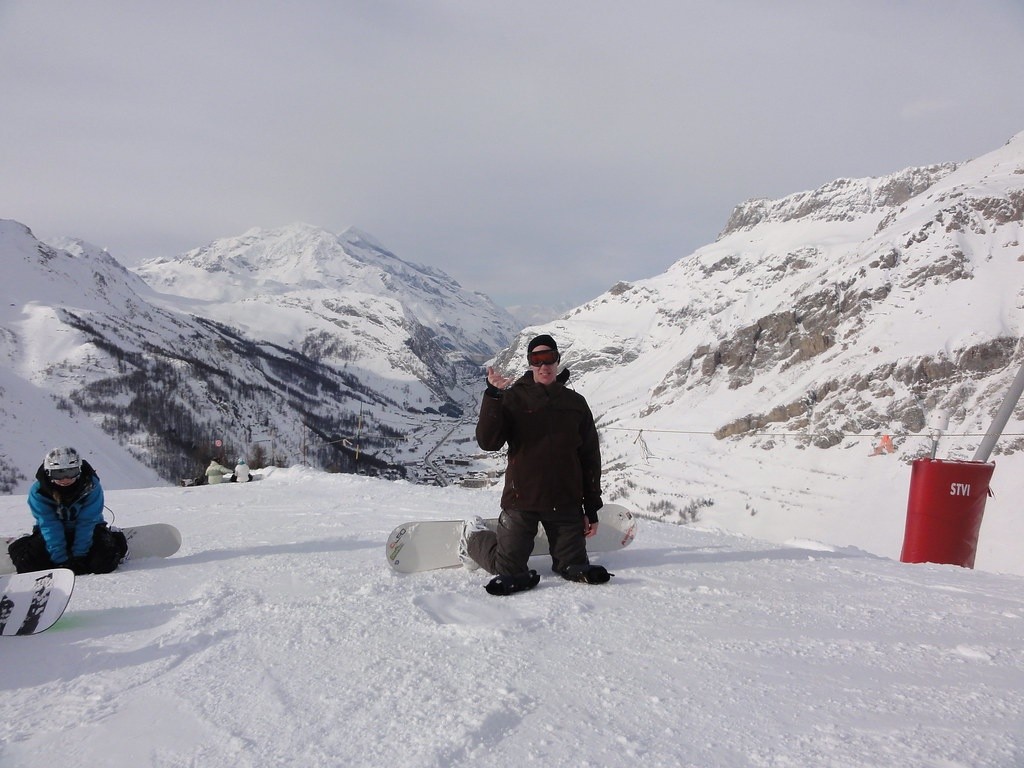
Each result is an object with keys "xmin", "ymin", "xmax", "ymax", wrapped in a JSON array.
[{"xmin": 70, "ymin": 554, "xmax": 89, "ymax": 574}]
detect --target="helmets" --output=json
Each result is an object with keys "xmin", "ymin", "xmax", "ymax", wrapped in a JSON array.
[{"xmin": 43, "ymin": 446, "xmax": 82, "ymax": 473}]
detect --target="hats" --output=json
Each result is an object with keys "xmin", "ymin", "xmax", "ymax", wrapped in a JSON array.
[
  {"xmin": 237, "ymin": 458, "xmax": 246, "ymax": 466},
  {"xmin": 213, "ymin": 457, "xmax": 221, "ymax": 464},
  {"xmin": 526, "ymin": 335, "xmax": 559, "ymax": 353}
]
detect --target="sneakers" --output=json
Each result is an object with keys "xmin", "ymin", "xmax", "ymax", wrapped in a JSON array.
[
  {"xmin": 457, "ymin": 514, "xmax": 484, "ymax": 573},
  {"xmin": 109, "ymin": 526, "xmax": 130, "ymax": 566},
  {"xmin": 5, "ymin": 533, "xmax": 31, "ymax": 552}
]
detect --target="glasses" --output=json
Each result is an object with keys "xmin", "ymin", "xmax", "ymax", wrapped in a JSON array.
[
  {"xmin": 527, "ymin": 349, "xmax": 561, "ymax": 367},
  {"xmin": 49, "ymin": 466, "xmax": 82, "ymax": 480}
]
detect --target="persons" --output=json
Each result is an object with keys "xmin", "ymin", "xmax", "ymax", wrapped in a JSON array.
[
  {"xmin": 6, "ymin": 446, "xmax": 130, "ymax": 575},
  {"xmin": 456, "ymin": 334, "xmax": 605, "ymax": 576}
]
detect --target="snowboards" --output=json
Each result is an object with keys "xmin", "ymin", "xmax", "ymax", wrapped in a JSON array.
[
  {"xmin": 384, "ymin": 505, "xmax": 638, "ymax": 574},
  {"xmin": 0, "ymin": 522, "xmax": 182, "ymax": 572},
  {"xmin": 226, "ymin": 474, "xmax": 262, "ymax": 483},
  {"xmin": 1, "ymin": 569, "xmax": 77, "ymax": 637}
]
